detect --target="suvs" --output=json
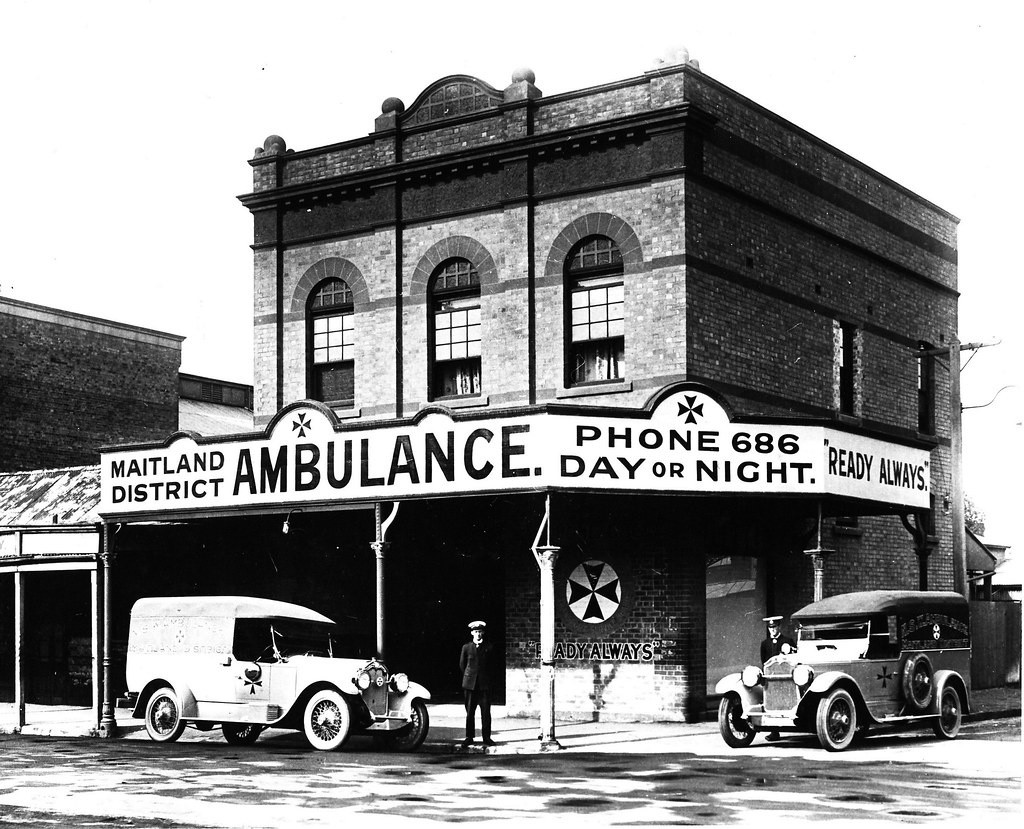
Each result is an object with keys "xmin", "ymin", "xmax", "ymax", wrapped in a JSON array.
[
  {"xmin": 125, "ymin": 596, "xmax": 432, "ymax": 753},
  {"xmin": 715, "ymin": 590, "xmax": 975, "ymax": 753}
]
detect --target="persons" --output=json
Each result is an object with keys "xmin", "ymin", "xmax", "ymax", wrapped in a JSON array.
[
  {"xmin": 460, "ymin": 620, "xmax": 496, "ymax": 749},
  {"xmin": 760, "ymin": 615, "xmax": 797, "ymax": 741}
]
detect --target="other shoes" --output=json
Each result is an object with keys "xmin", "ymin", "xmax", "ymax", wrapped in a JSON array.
[
  {"xmin": 766, "ymin": 731, "xmax": 779, "ymax": 742},
  {"xmin": 483, "ymin": 737, "xmax": 495, "ymax": 747},
  {"xmin": 462, "ymin": 738, "xmax": 473, "ymax": 748}
]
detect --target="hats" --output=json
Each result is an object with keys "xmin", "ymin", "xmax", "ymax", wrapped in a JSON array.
[
  {"xmin": 762, "ymin": 616, "xmax": 784, "ymax": 629},
  {"xmin": 468, "ymin": 621, "xmax": 487, "ymax": 631}
]
